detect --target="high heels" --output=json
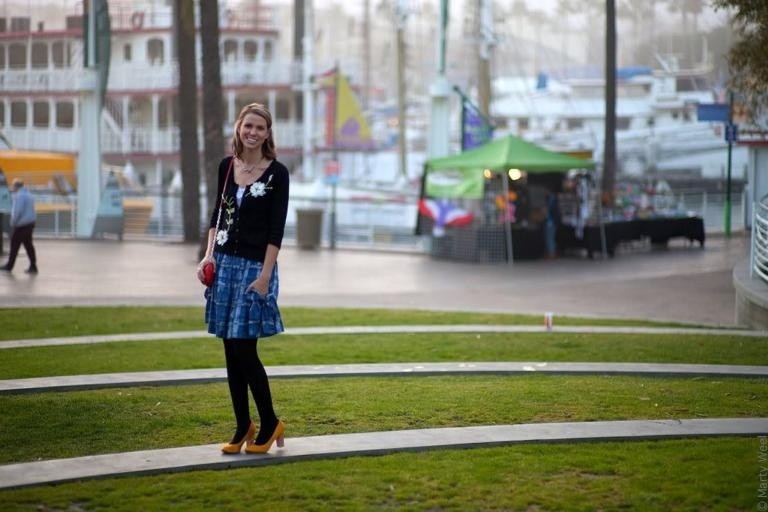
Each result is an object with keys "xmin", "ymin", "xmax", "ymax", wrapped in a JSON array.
[
  {"xmin": 245, "ymin": 419, "xmax": 284, "ymax": 453},
  {"xmin": 222, "ymin": 420, "xmax": 257, "ymax": 453}
]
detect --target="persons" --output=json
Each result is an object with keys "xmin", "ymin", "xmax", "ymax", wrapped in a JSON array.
[
  {"xmin": 0, "ymin": 174, "xmax": 12, "ymax": 257},
  {"xmin": 540, "ymin": 184, "xmax": 562, "ymax": 261},
  {"xmin": 0, "ymin": 176, "xmax": 39, "ymax": 274},
  {"xmin": 196, "ymin": 102, "xmax": 290, "ymax": 455}
]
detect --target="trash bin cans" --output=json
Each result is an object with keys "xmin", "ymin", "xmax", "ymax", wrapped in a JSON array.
[{"xmin": 296, "ymin": 209, "xmax": 324, "ymax": 251}]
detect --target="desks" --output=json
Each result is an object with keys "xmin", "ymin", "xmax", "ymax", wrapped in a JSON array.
[{"xmin": 576, "ymin": 215, "xmax": 706, "ymax": 263}]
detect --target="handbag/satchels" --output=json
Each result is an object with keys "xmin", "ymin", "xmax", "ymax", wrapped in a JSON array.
[{"xmin": 197, "ymin": 257, "xmax": 215, "ymax": 286}]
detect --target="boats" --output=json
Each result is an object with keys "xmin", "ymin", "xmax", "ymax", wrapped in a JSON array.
[
  {"xmin": 0, "ymin": 0, "xmax": 315, "ymax": 184},
  {"xmin": 33, "ymin": 197, "xmax": 155, "ymax": 235},
  {"xmin": 464, "ymin": 61, "xmax": 749, "ymax": 183},
  {"xmin": 284, "ymin": 181, "xmax": 419, "ymax": 237},
  {"xmin": 0, "ymin": 133, "xmax": 128, "ymax": 196}
]
